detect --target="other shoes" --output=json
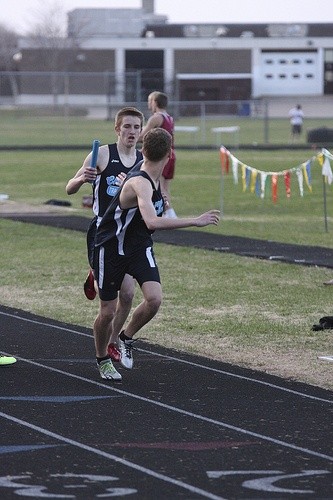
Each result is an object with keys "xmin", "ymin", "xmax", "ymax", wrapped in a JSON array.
[
  {"xmin": 162, "ymin": 214, "xmax": 178, "ymax": 219},
  {"xmin": 0, "ymin": 356, "xmax": 17, "ymax": 365}
]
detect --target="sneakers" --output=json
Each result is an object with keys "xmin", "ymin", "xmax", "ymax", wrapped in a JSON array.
[
  {"xmin": 84, "ymin": 271, "xmax": 97, "ymax": 299},
  {"xmin": 96, "ymin": 360, "xmax": 122, "ymax": 380},
  {"xmin": 118, "ymin": 335, "xmax": 139, "ymax": 369},
  {"xmin": 109, "ymin": 344, "xmax": 121, "ymax": 361}
]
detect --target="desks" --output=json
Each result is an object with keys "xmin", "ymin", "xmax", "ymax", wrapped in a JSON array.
[
  {"xmin": 211, "ymin": 127, "xmax": 240, "ymax": 148},
  {"xmin": 173, "ymin": 126, "xmax": 199, "ymax": 146}
]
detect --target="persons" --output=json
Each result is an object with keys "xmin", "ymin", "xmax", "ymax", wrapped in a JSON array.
[
  {"xmin": 93, "ymin": 127, "xmax": 221, "ymax": 380},
  {"xmin": 66, "ymin": 107, "xmax": 144, "ymax": 300},
  {"xmin": 290, "ymin": 103, "xmax": 305, "ymax": 142},
  {"xmin": 139, "ymin": 91, "xmax": 176, "ymax": 195}
]
